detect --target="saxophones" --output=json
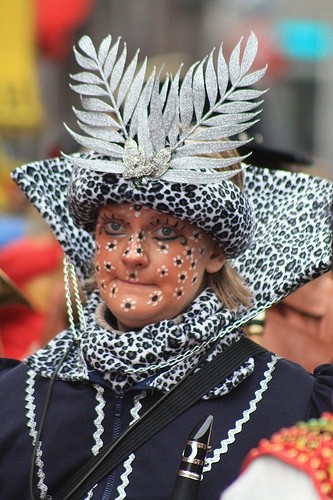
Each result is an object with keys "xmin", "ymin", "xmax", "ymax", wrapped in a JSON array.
[{"xmin": 170, "ymin": 415, "xmax": 215, "ymax": 500}]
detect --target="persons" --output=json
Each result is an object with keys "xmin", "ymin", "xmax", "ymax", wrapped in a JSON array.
[{"xmin": 1, "ymin": 31, "xmax": 333, "ymax": 500}]
